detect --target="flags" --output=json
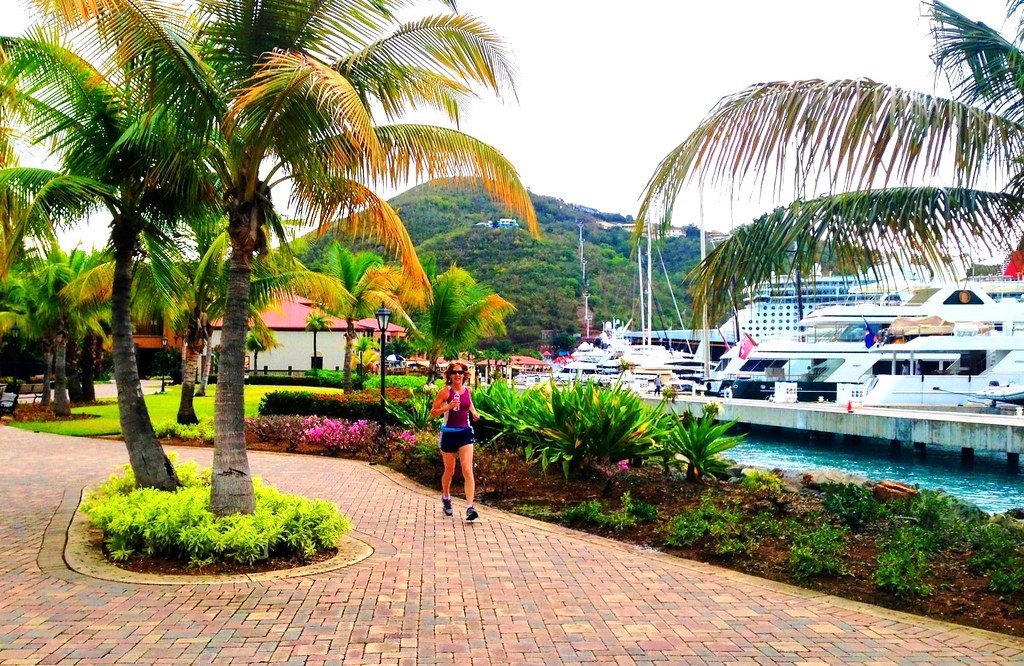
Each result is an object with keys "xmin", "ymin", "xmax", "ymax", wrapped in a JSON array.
[
  {"xmin": 738, "ymin": 332, "xmax": 758, "ymax": 360},
  {"xmin": 861, "ymin": 315, "xmax": 879, "ymax": 349}
]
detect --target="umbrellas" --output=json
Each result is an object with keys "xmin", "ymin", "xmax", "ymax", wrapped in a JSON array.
[{"xmin": 386, "ymin": 354, "xmax": 406, "ymax": 367}]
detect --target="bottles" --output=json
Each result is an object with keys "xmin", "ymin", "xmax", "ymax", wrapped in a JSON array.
[{"xmin": 453, "ymin": 392, "xmax": 460, "ymax": 411}]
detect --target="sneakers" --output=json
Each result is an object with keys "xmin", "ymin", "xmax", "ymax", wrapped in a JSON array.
[
  {"xmin": 441, "ymin": 496, "xmax": 453, "ymax": 516},
  {"xmin": 465, "ymin": 507, "xmax": 478, "ymax": 520}
]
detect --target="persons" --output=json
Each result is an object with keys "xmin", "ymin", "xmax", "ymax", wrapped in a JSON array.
[
  {"xmin": 901, "ymin": 358, "xmax": 930, "ymax": 375},
  {"xmin": 430, "ymin": 362, "xmax": 481, "ymax": 520},
  {"xmin": 707, "ymin": 382, "xmax": 711, "ymax": 393},
  {"xmin": 653, "ymin": 373, "xmax": 662, "ymax": 396}
]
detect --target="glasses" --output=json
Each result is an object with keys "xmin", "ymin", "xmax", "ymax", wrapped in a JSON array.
[{"xmin": 450, "ymin": 370, "xmax": 465, "ymax": 374}]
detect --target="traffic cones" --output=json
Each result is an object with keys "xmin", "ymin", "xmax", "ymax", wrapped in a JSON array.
[{"xmin": 845, "ymin": 402, "xmax": 852, "ymax": 414}]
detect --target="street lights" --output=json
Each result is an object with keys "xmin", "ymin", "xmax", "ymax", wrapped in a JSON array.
[
  {"xmin": 374, "ymin": 301, "xmax": 391, "ymax": 450},
  {"xmin": 160, "ymin": 336, "xmax": 168, "ymax": 392}
]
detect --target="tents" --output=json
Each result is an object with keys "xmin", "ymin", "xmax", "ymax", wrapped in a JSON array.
[
  {"xmin": 887, "ymin": 316, "xmax": 955, "ymax": 343},
  {"xmin": 845, "ymin": 276, "xmax": 932, "ymax": 306}
]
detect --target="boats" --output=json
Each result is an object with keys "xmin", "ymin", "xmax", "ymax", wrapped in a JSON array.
[{"xmin": 509, "ymin": 176, "xmax": 1024, "ymax": 409}]
